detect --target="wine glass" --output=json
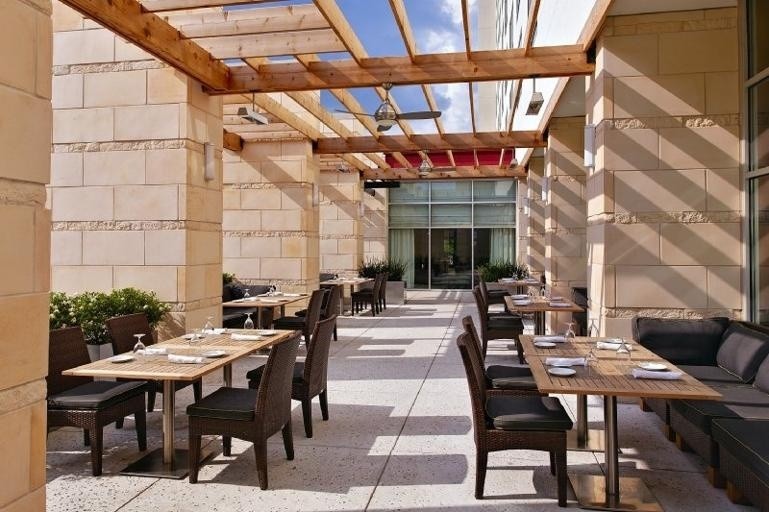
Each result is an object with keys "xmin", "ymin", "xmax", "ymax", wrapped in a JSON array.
[
  {"xmin": 563, "ymin": 317, "xmax": 633, "ymax": 378},
  {"xmin": 132, "ymin": 312, "xmax": 254, "ymax": 359},
  {"xmin": 243, "ymin": 284, "xmax": 281, "ymax": 302},
  {"xmin": 510, "ymin": 269, "xmax": 551, "ymax": 307}
]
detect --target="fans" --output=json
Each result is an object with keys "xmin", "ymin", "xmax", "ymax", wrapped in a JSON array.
[
  {"xmin": 335, "ymin": 81, "xmax": 441, "ymax": 132},
  {"xmin": 393, "ymin": 150, "xmax": 457, "ymax": 175}
]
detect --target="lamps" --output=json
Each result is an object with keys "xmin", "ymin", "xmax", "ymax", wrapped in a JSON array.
[
  {"xmin": 338, "ymin": 159, "xmax": 351, "ymax": 174},
  {"xmin": 237, "ymin": 94, "xmax": 269, "ymax": 125},
  {"xmin": 509, "ymin": 148, "xmax": 518, "ymax": 170},
  {"xmin": 526, "ymin": 78, "xmax": 544, "ymax": 116}
]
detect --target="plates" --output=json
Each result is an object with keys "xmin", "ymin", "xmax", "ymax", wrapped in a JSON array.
[
  {"xmin": 548, "ymin": 367, "xmax": 576, "ymax": 375},
  {"xmin": 534, "ymin": 341, "xmax": 557, "ymax": 347},
  {"xmin": 258, "ymin": 329, "xmax": 276, "ymax": 335},
  {"xmin": 638, "ymin": 361, "xmax": 667, "ymax": 372},
  {"xmin": 109, "ymin": 355, "xmax": 133, "ymax": 363}
]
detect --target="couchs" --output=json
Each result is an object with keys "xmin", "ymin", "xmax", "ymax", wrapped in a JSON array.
[{"xmin": 631, "ymin": 316, "xmax": 769, "ymax": 511}]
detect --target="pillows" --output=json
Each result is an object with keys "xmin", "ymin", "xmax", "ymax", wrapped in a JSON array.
[
  {"xmin": 716, "ymin": 321, "xmax": 769, "ymax": 384},
  {"xmin": 754, "ymin": 355, "xmax": 769, "ymax": 394}
]
